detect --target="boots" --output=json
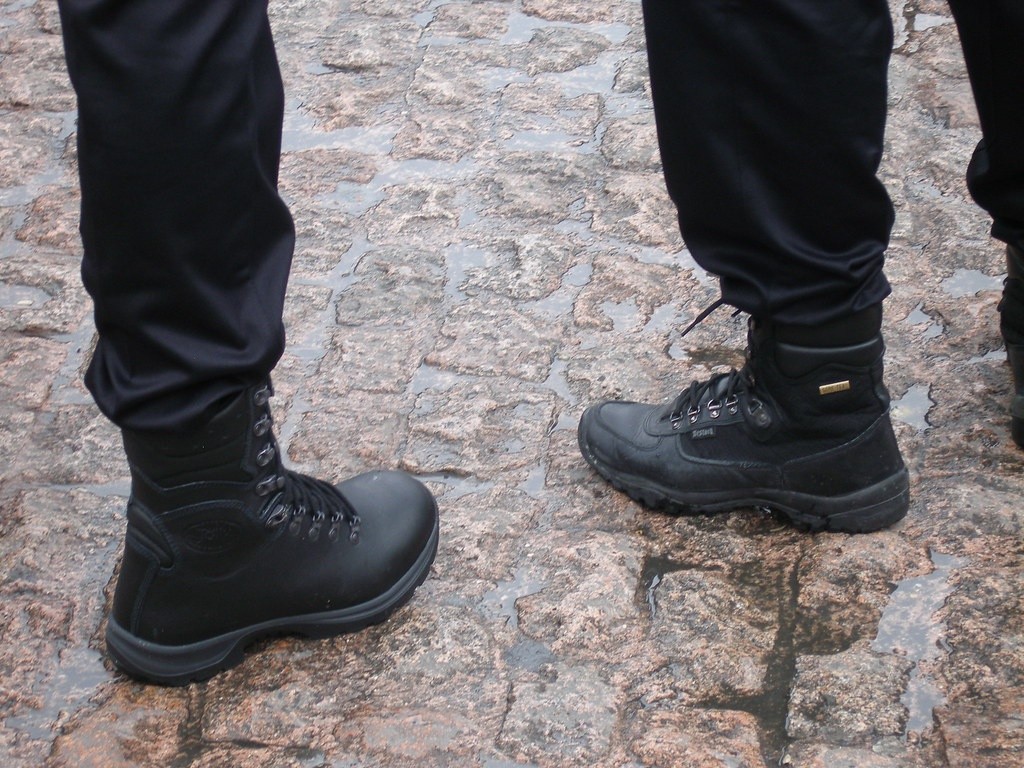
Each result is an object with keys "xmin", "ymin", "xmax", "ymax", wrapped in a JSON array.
[
  {"xmin": 106, "ymin": 381, "xmax": 441, "ymax": 681},
  {"xmin": 578, "ymin": 342, "xmax": 909, "ymax": 541}
]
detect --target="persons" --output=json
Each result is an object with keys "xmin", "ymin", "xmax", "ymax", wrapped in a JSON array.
[
  {"xmin": 578, "ymin": 0, "xmax": 1024, "ymax": 533},
  {"xmin": 52, "ymin": 0, "xmax": 441, "ymax": 686}
]
